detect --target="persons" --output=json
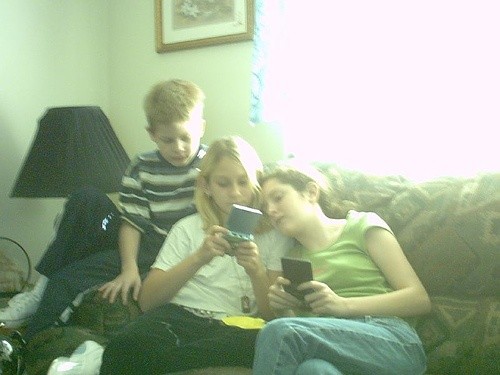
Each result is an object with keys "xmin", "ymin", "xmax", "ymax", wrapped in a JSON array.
[
  {"xmin": 0, "ymin": 78, "xmax": 211, "ymax": 330},
  {"xmin": 252, "ymin": 167, "xmax": 432, "ymax": 375},
  {"xmin": 99, "ymin": 135, "xmax": 295, "ymax": 375}
]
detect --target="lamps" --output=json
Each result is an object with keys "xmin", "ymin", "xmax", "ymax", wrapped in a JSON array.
[{"xmin": 8, "ymin": 104, "xmax": 131, "ymax": 199}]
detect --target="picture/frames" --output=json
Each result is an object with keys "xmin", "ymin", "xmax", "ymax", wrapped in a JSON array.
[{"xmin": 154, "ymin": 0, "xmax": 254, "ymax": 54}]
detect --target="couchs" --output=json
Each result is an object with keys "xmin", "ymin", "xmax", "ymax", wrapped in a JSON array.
[{"xmin": 24, "ymin": 172, "xmax": 500, "ymax": 375}]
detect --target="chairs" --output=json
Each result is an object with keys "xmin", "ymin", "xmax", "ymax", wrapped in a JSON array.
[{"xmin": 0, "ymin": 237, "xmax": 31, "ymax": 335}]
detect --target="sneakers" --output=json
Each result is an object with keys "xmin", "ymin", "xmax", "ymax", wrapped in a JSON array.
[
  {"xmin": 0, "ymin": 292, "xmax": 41, "ymax": 327},
  {"xmin": 48, "ymin": 340, "xmax": 104, "ymax": 375}
]
